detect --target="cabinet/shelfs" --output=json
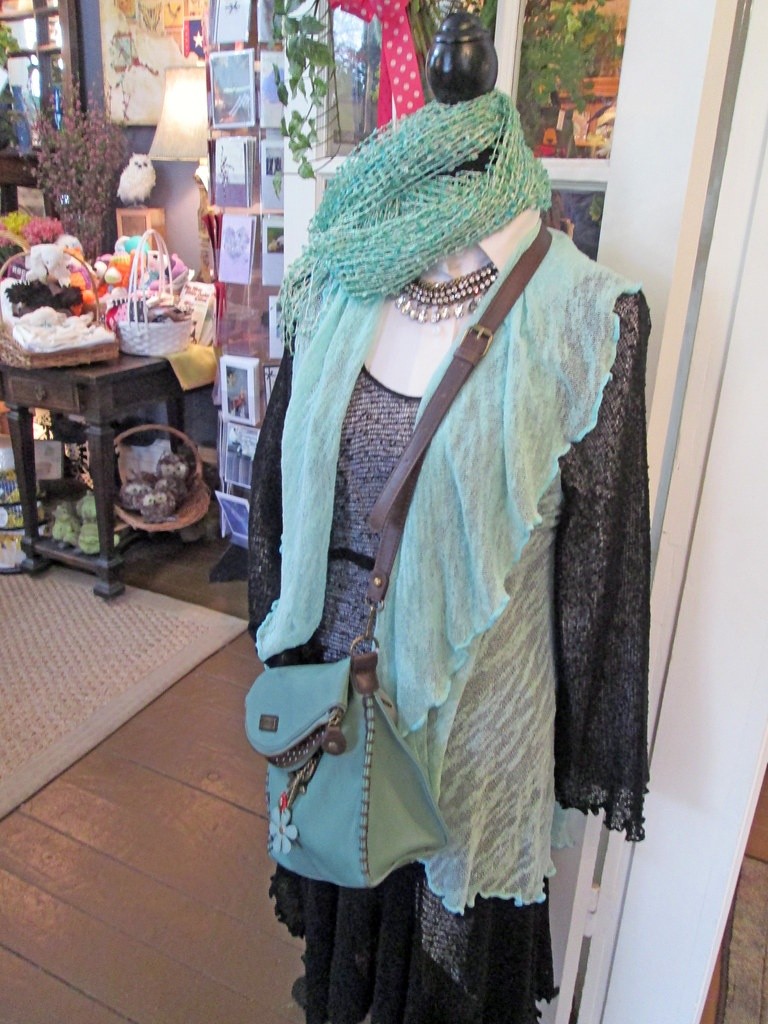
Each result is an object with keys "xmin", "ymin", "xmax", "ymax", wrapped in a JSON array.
[{"xmin": 0, "ymin": 353, "xmax": 211, "ymax": 599}]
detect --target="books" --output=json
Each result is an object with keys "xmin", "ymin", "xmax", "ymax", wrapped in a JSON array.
[{"xmin": 178, "ymin": 282, "xmax": 215, "ymax": 345}]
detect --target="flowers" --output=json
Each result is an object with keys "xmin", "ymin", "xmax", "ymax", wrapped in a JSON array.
[
  {"xmin": 0, "ymin": 208, "xmax": 63, "ymax": 282},
  {"xmin": 9, "ymin": 64, "xmax": 132, "ymax": 268}
]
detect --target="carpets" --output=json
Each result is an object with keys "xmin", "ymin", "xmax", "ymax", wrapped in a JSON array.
[{"xmin": 0, "ymin": 564, "xmax": 251, "ymax": 821}]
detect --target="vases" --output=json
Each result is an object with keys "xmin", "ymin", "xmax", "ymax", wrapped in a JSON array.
[{"xmin": 62, "ymin": 213, "xmax": 105, "ymax": 268}]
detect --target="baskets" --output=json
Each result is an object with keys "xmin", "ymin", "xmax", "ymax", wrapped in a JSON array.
[
  {"xmin": 111, "ymin": 228, "xmax": 194, "ymax": 356},
  {"xmin": 108, "ymin": 423, "xmax": 212, "ymax": 532},
  {"xmin": 0, "ymin": 249, "xmax": 119, "ymax": 372}
]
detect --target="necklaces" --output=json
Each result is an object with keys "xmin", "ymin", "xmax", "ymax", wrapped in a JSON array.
[{"xmin": 396, "ymin": 263, "xmax": 499, "ymax": 325}]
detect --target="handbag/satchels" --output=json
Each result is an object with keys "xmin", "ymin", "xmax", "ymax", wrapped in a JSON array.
[{"xmin": 244, "ymin": 635, "xmax": 452, "ymax": 891}]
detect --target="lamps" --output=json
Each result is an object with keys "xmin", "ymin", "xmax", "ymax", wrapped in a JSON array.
[{"xmin": 146, "ymin": 66, "xmax": 215, "ymax": 285}]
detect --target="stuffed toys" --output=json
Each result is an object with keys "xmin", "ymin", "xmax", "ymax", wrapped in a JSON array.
[
  {"xmin": 0, "ymin": 235, "xmax": 194, "ymax": 354},
  {"xmin": 116, "ymin": 153, "xmax": 156, "ymax": 205},
  {"xmin": 123, "ymin": 454, "xmax": 188, "ymax": 523},
  {"xmin": 50, "ymin": 490, "xmax": 120, "ymax": 554}
]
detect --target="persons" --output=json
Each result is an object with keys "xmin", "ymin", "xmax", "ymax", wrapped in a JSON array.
[{"xmin": 245, "ymin": 96, "xmax": 653, "ymax": 1024}]
